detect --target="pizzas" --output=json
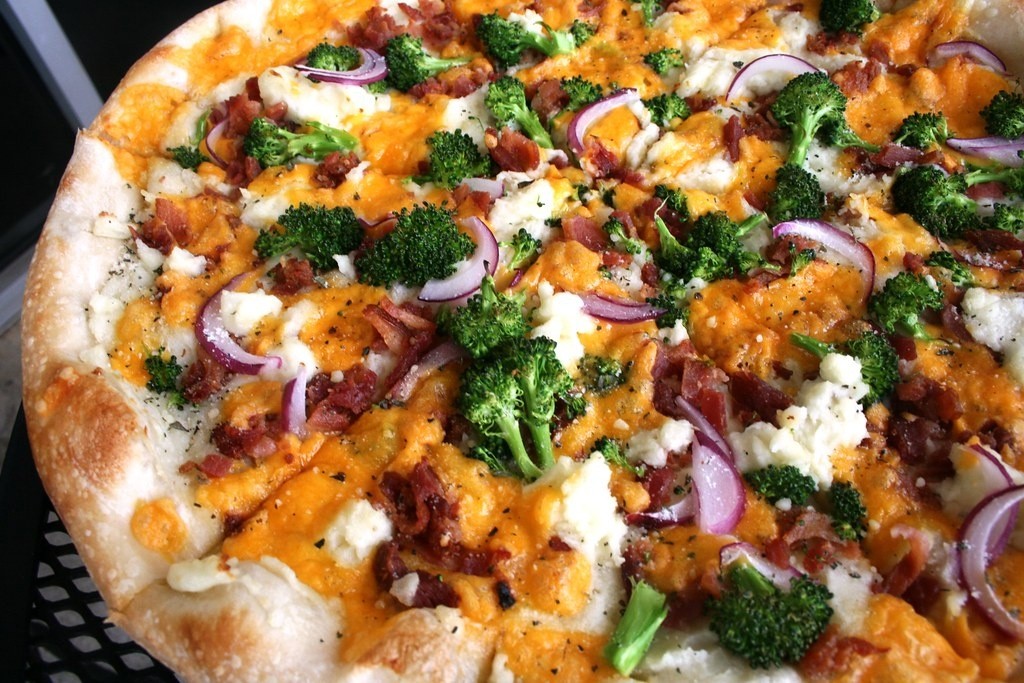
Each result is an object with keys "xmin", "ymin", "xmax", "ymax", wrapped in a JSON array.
[{"xmin": 20, "ymin": 0, "xmax": 1024, "ymax": 683}]
